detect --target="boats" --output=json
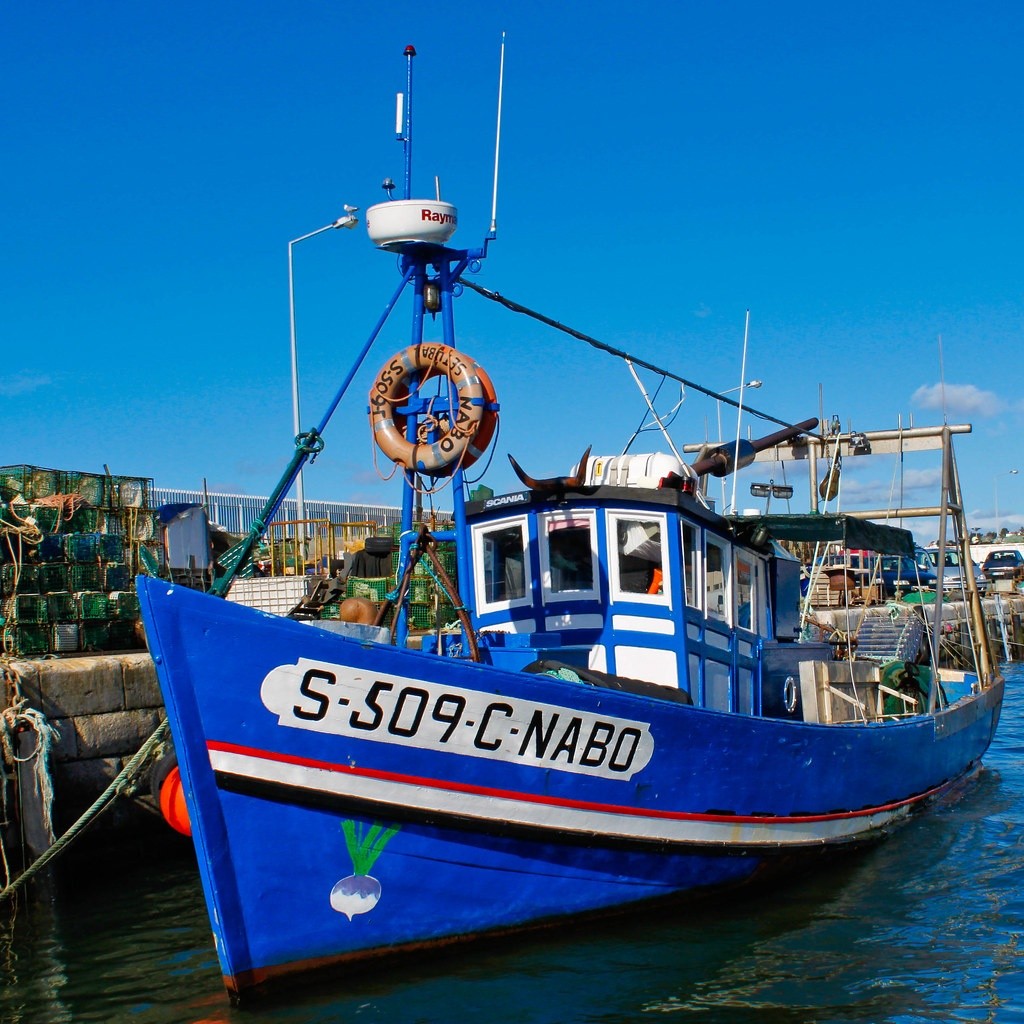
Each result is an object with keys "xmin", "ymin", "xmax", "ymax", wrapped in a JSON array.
[{"xmin": 135, "ymin": 34, "xmax": 1002, "ymax": 1004}]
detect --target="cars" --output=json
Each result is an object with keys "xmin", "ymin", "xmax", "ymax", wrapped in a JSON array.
[
  {"xmin": 980, "ymin": 550, "xmax": 1024, "ymax": 583},
  {"xmin": 800, "ymin": 554, "xmax": 937, "ymax": 596},
  {"xmin": 923, "ymin": 547, "xmax": 982, "ymax": 577}
]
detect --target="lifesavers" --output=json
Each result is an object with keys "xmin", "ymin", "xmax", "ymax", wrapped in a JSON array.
[{"xmin": 367, "ymin": 341, "xmax": 499, "ymax": 479}]
